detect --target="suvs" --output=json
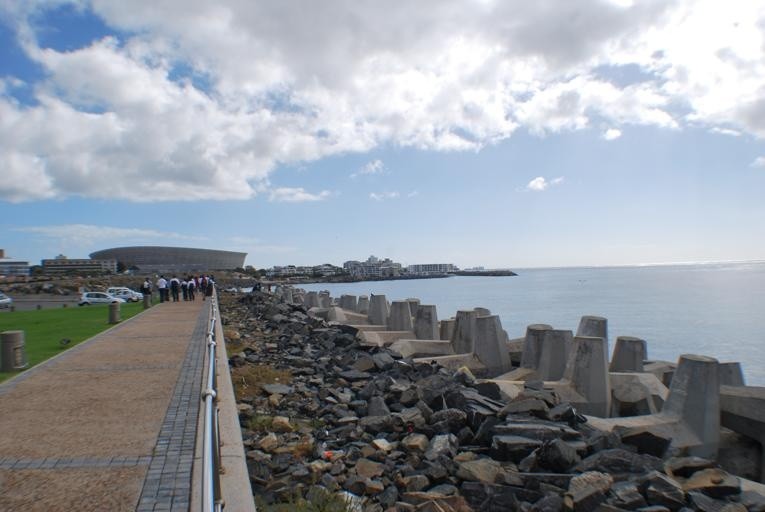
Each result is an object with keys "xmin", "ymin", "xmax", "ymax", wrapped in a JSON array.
[{"xmin": 78, "ymin": 285, "xmax": 144, "ymax": 305}]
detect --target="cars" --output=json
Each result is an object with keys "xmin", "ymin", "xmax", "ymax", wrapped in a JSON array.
[{"xmin": 0, "ymin": 293, "xmax": 12, "ymax": 310}]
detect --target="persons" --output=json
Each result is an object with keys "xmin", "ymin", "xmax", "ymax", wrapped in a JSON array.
[
  {"xmin": 140, "ymin": 273, "xmax": 216, "ymax": 310},
  {"xmin": 252, "ymin": 282, "xmax": 261, "ymax": 292}
]
